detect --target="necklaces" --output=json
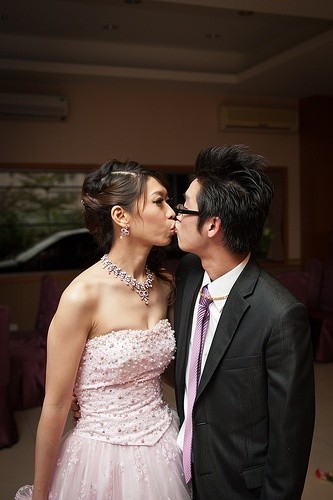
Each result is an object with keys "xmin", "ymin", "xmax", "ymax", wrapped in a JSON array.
[{"xmin": 100, "ymin": 253, "xmax": 154, "ymax": 307}]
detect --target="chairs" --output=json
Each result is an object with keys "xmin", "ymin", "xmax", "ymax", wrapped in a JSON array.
[{"xmin": 0, "ymin": 256, "xmax": 333, "ymax": 449}]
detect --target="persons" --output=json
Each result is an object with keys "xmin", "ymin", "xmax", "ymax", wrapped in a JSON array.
[
  {"xmin": 66, "ymin": 142, "xmax": 314, "ymax": 500},
  {"xmin": 31, "ymin": 160, "xmax": 191, "ymax": 500}
]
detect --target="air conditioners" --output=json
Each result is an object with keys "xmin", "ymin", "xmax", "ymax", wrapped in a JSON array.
[
  {"xmin": 219, "ymin": 105, "xmax": 299, "ymax": 135},
  {"xmin": 0, "ymin": 91, "xmax": 68, "ymax": 123}
]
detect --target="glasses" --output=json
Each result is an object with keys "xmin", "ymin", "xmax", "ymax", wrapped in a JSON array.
[{"xmin": 175, "ymin": 203, "xmax": 199, "ymax": 216}]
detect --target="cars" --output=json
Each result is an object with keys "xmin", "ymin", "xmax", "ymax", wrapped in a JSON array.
[{"xmin": 0, "ymin": 228, "xmax": 95, "ymax": 275}]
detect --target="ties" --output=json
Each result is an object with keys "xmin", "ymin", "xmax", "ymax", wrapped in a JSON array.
[{"xmin": 182, "ymin": 286, "xmax": 214, "ymax": 484}]
